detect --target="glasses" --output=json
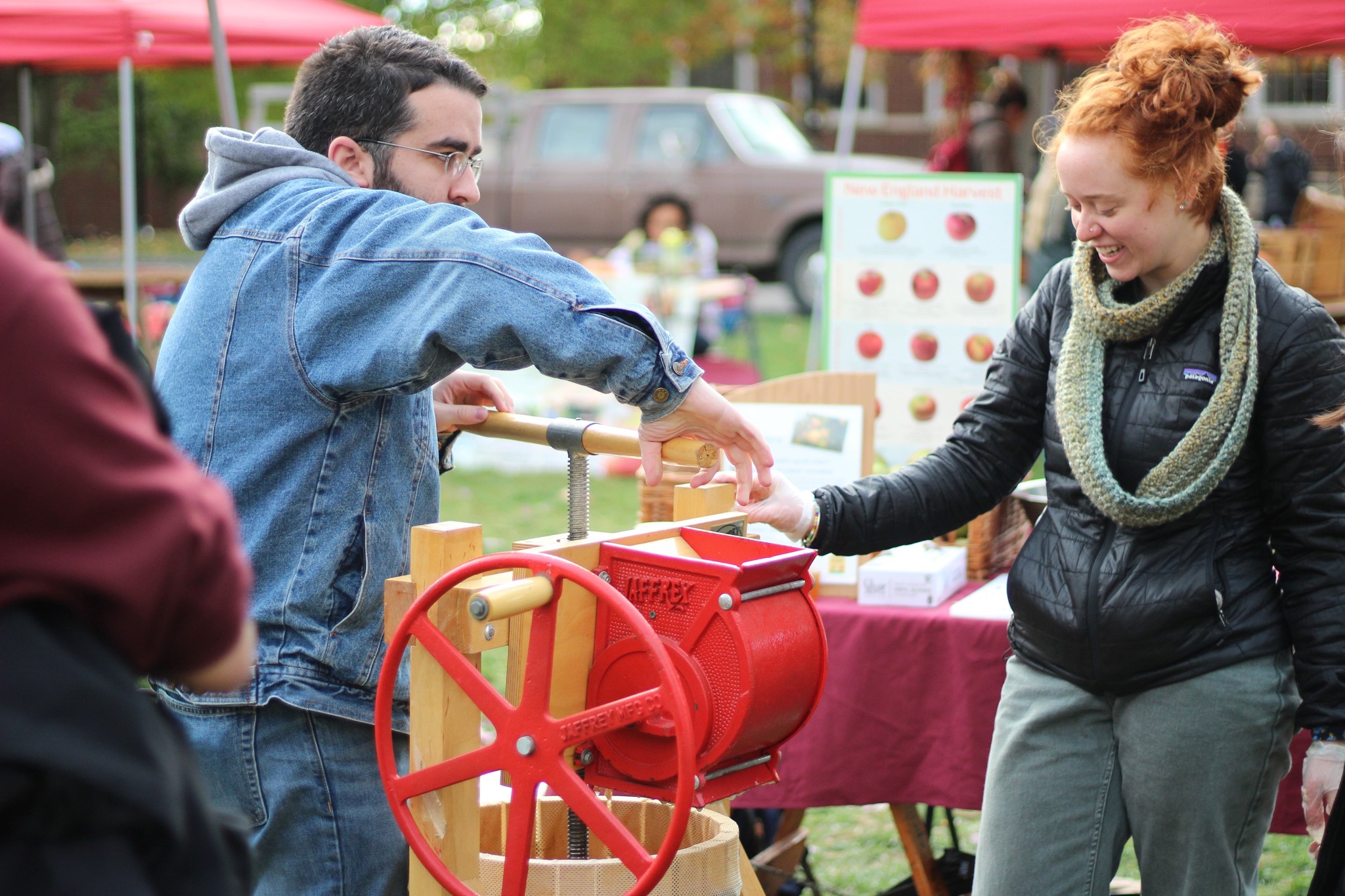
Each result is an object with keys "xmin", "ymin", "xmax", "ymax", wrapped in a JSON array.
[{"xmin": 354, "ymin": 138, "xmax": 483, "ymax": 185}]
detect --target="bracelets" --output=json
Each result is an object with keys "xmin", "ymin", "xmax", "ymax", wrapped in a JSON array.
[{"xmin": 802, "ymin": 504, "xmax": 820, "ymax": 546}]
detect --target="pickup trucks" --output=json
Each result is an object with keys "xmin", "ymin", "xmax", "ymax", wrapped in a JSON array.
[{"xmin": 454, "ymin": 86, "xmax": 930, "ymax": 320}]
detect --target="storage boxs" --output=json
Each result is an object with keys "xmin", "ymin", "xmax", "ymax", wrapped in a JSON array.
[{"xmin": 857, "ymin": 545, "xmax": 968, "ymax": 608}]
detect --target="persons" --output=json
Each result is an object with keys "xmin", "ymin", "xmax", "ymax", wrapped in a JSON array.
[
  {"xmin": 710, "ymin": 12, "xmax": 1345, "ymax": 896},
  {"xmin": 0, "ymin": 224, "xmax": 260, "ymax": 896},
  {"xmin": 1218, "ymin": 122, "xmax": 1246, "ymax": 196},
  {"xmin": 0, "ymin": 122, "xmax": 67, "ymax": 259},
  {"xmin": 1020, "ymin": 145, "xmax": 1077, "ymax": 295},
  {"xmin": 930, "ymin": 72, "xmax": 1030, "ymax": 172},
  {"xmin": 145, "ymin": 26, "xmax": 773, "ymax": 896},
  {"xmin": 613, "ymin": 194, "xmax": 721, "ymax": 361},
  {"xmin": 1248, "ymin": 115, "xmax": 1312, "ymax": 225}
]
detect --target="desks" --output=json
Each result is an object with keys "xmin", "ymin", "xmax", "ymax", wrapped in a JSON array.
[{"xmin": 728, "ymin": 565, "xmax": 1312, "ymax": 896}]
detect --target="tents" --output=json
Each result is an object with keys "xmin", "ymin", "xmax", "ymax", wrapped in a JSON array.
[
  {"xmin": 0, "ymin": 0, "xmax": 395, "ymax": 349},
  {"xmin": 806, "ymin": 0, "xmax": 1345, "ymax": 377}
]
